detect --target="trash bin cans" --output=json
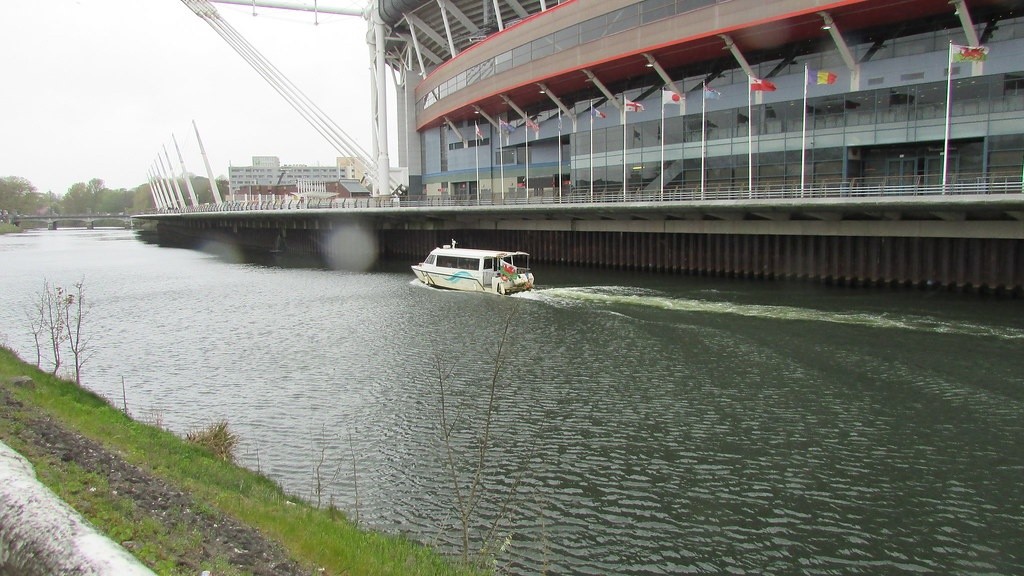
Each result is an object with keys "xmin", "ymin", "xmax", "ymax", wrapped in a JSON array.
[{"xmin": 390, "ymin": 196, "xmax": 400, "ymax": 208}]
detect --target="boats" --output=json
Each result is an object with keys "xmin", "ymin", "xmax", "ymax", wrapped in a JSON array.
[{"xmin": 410, "ymin": 239, "xmax": 535, "ymax": 296}]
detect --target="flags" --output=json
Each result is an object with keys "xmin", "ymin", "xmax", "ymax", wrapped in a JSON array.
[
  {"xmin": 750, "ymin": 78, "xmax": 776, "ymax": 91},
  {"xmin": 806, "ymin": 69, "xmax": 837, "ymax": 85},
  {"xmin": 500, "ymin": 260, "xmax": 517, "ymax": 280},
  {"xmin": 558, "ymin": 118, "xmax": 562, "ymax": 131},
  {"xmin": 476, "ymin": 124, "xmax": 483, "ymax": 141},
  {"xmin": 592, "ymin": 107, "xmax": 606, "ymax": 119},
  {"xmin": 663, "ymin": 91, "xmax": 681, "ymax": 104},
  {"xmin": 951, "ymin": 44, "xmax": 990, "ymax": 62},
  {"xmin": 625, "ymin": 99, "xmax": 645, "ymax": 111},
  {"xmin": 500, "ymin": 120, "xmax": 516, "ymax": 131},
  {"xmin": 704, "ymin": 86, "xmax": 721, "ymax": 96},
  {"xmin": 527, "ymin": 118, "xmax": 539, "ymax": 132}
]
